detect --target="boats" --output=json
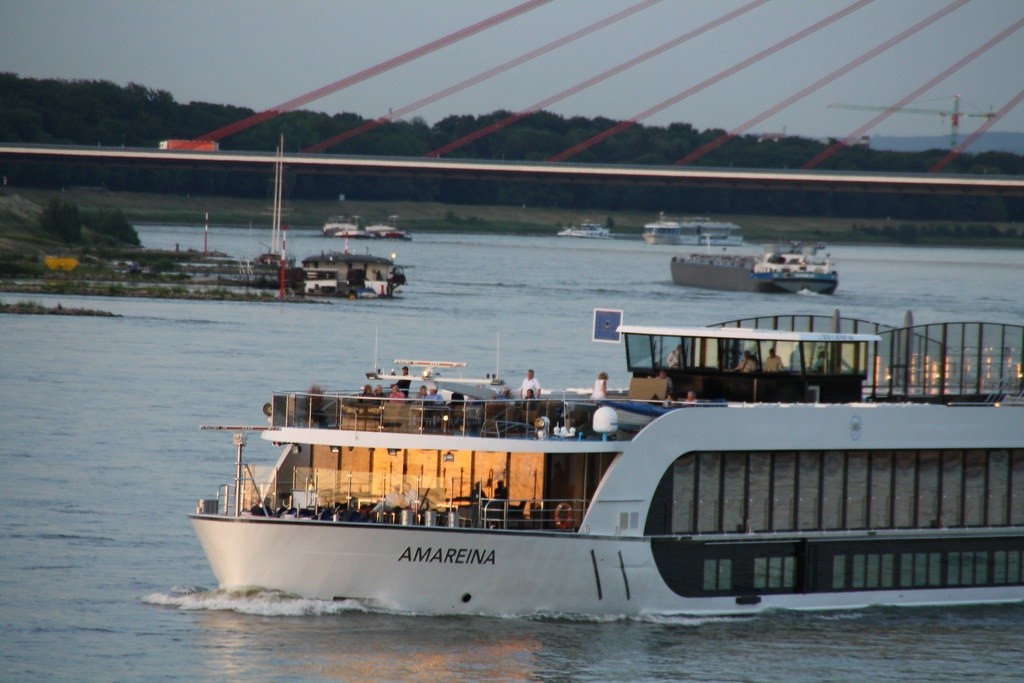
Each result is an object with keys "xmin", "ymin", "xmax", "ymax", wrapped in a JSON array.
[
  {"xmin": 323, "ymin": 214, "xmax": 413, "ymax": 242},
  {"xmin": 670, "ymin": 241, "xmax": 838, "ymax": 296},
  {"xmin": 644, "ymin": 218, "xmax": 741, "ymax": 248},
  {"xmin": 188, "ymin": 309, "xmax": 1024, "ymax": 619},
  {"xmin": 302, "ymin": 251, "xmax": 406, "ymax": 298},
  {"xmin": 560, "ymin": 220, "xmax": 610, "ymax": 239}
]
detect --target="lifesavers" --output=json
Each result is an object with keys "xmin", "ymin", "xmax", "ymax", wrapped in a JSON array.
[{"xmin": 555, "ymin": 502, "xmax": 573, "ymax": 528}]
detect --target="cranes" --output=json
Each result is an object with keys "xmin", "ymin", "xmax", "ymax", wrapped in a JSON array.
[{"xmin": 826, "ymin": 93, "xmax": 995, "ymax": 151}]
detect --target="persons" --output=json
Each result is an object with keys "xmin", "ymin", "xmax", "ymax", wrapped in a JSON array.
[
  {"xmin": 811, "ymin": 351, "xmax": 825, "ymax": 374},
  {"xmin": 589, "ymin": 371, "xmax": 608, "ymax": 401},
  {"xmin": 360, "ymin": 367, "xmax": 541, "ymax": 435},
  {"xmin": 368, "ymin": 479, "xmax": 508, "ymax": 528},
  {"xmin": 764, "ymin": 349, "xmax": 784, "ymax": 371},
  {"xmin": 305, "ymin": 386, "xmax": 329, "ymax": 428},
  {"xmin": 725, "ymin": 351, "xmax": 757, "ymax": 372},
  {"xmin": 647, "ymin": 345, "xmax": 698, "ymax": 403}
]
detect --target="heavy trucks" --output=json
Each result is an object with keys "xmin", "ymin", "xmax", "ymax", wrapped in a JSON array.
[{"xmin": 159, "ymin": 140, "xmax": 219, "ymax": 151}]
